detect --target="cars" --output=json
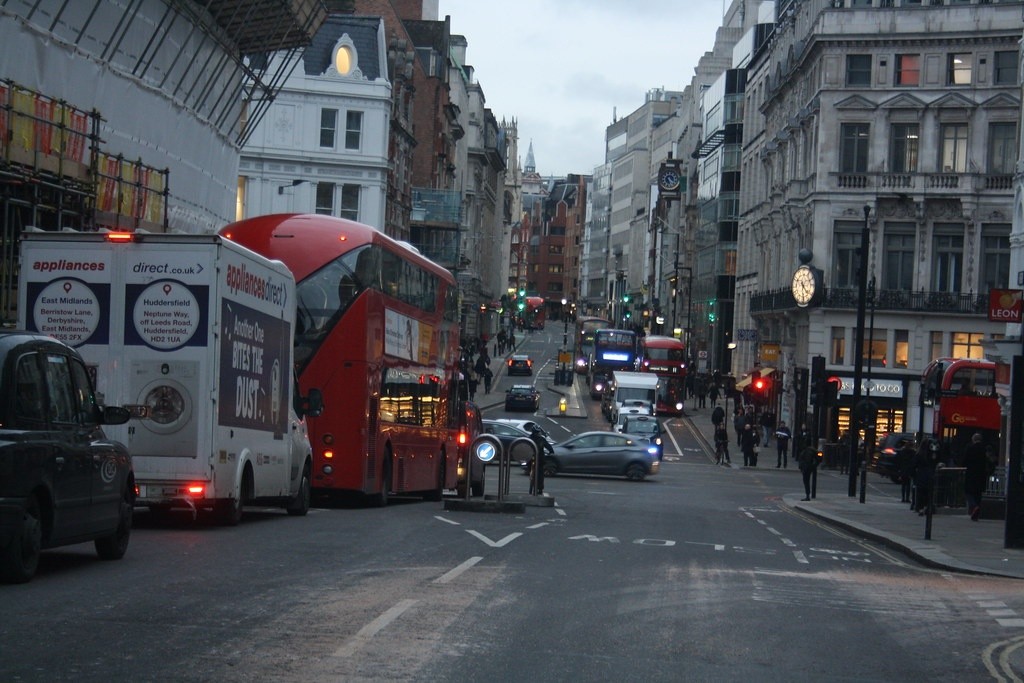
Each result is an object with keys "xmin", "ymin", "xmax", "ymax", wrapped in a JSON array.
[
  {"xmin": 521, "ymin": 431, "xmax": 662, "ymax": 480},
  {"xmin": 504, "ymin": 385, "xmax": 542, "ymax": 408},
  {"xmin": 480, "ymin": 422, "xmax": 540, "ymax": 462},
  {"xmin": 612, "ymin": 397, "xmax": 667, "ymax": 458},
  {"xmin": 0, "ymin": 331, "xmax": 135, "ymax": 586},
  {"xmin": 507, "ymin": 354, "xmax": 535, "ymax": 375},
  {"xmin": 457, "ymin": 401, "xmax": 484, "ymax": 466},
  {"xmin": 495, "ymin": 418, "xmax": 558, "ymax": 444}
]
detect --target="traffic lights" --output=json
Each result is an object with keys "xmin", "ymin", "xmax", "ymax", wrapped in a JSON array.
[
  {"xmin": 810, "ymin": 378, "xmax": 824, "ymax": 406},
  {"xmin": 753, "ymin": 378, "xmax": 769, "ymax": 407}
]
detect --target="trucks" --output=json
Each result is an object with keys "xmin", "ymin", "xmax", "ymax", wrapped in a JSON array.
[{"xmin": 15, "ymin": 223, "xmax": 326, "ymax": 533}]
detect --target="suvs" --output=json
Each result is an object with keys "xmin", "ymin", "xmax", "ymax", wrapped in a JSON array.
[{"xmin": 877, "ymin": 431, "xmax": 945, "ymax": 484}]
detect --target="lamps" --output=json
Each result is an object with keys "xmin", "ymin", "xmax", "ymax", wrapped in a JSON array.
[
  {"xmin": 728, "ymin": 343, "xmax": 736, "ymax": 350},
  {"xmin": 279, "ymin": 180, "xmax": 311, "ymax": 193}
]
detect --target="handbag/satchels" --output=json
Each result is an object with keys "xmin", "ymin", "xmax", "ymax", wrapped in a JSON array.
[{"xmin": 752, "ymin": 445, "xmax": 762, "ymax": 453}]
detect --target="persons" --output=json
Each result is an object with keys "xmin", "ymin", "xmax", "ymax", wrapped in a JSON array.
[
  {"xmin": 483, "ymin": 366, "xmax": 493, "ymax": 394},
  {"xmin": 760, "ymin": 411, "xmax": 770, "ymax": 447},
  {"xmin": 687, "ymin": 369, "xmax": 736, "ymax": 408},
  {"xmin": 913, "ymin": 437, "xmax": 945, "ymax": 514},
  {"xmin": 715, "ymin": 403, "xmax": 725, "ymax": 425},
  {"xmin": 798, "ymin": 439, "xmax": 818, "ymax": 501},
  {"xmin": 897, "ymin": 442, "xmax": 916, "ymax": 504},
  {"xmin": 740, "ymin": 424, "xmax": 760, "ymax": 466},
  {"xmin": 840, "ymin": 431, "xmax": 851, "ymax": 474},
  {"xmin": 714, "ymin": 422, "xmax": 731, "ymax": 463},
  {"xmin": 733, "ymin": 400, "xmax": 752, "ymax": 447},
  {"xmin": 529, "ymin": 424, "xmax": 554, "ymax": 494},
  {"xmin": 498, "ymin": 331, "xmax": 516, "ymax": 353},
  {"xmin": 962, "ymin": 433, "xmax": 990, "ymax": 522},
  {"xmin": 775, "ymin": 421, "xmax": 791, "ymax": 468},
  {"xmin": 469, "ymin": 371, "xmax": 479, "ymax": 401},
  {"xmin": 477, "ymin": 353, "xmax": 485, "ymax": 384},
  {"xmin": 798, "ymin": 424, "xmax": 809, "ymax": 450}
]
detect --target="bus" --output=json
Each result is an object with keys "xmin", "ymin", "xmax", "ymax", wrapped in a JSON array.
[
  {"xmin": 574, "ymin": 316, "xmax": 621, "ymax": 372},
  {"xmin": 585, "ymin": 328, "xmax": 646, "ymax": 400},
  {"xmin": 212, "ymin": 215, "xmax": 471, "ymax": 508},
  {"xmin": 638, "ymin": 335, "xmax": 690, "ymax": 416},
  {"xmin": 919, "ymin": 355, "xmax": 1004, "ymax": 459},
  {"xmin": 522, "ymin": 297, "xmax": 548, "ymax": 330}
]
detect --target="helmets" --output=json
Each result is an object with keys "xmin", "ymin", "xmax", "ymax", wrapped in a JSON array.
[{"xmin": 532, "ymin": 425, "xmax": 541, "ymax": 435}]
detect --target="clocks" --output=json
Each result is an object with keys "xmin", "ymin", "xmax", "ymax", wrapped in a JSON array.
[
  {"xmin": 791, "ymin": 249, "xmax": 824, "ymax": 308},
  {"xmin": 658, "ymin": 163, "xmax": 683, "ymax": 200}
]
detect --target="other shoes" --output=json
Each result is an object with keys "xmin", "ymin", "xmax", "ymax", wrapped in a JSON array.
[
  {"xmin": 919, "ymin": 512, "xmax": 924, "ymax": 516},
  {"xmin": 972, "ymin": 505, "xmax": 979, "ymax": 521}
]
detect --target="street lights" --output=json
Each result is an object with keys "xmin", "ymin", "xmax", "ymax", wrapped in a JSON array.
[{"xmin": 561, "ymin": 298, "xmax": 572, "ymax": 351}]
